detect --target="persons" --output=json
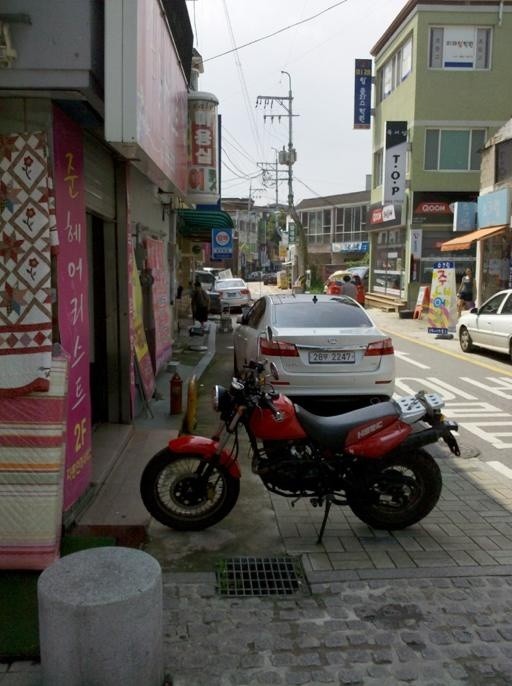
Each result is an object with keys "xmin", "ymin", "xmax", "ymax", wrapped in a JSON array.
[
  {"xmin": 456, "ymin": 267, "xmax": 475, "ymax": 318},
  {"xmin": 190, "ymin": 281, "xmax": 209, "ymax": 326},
  {"xmin": 340, "ymin": 275, "xmax": 358, "ymax": 300}
]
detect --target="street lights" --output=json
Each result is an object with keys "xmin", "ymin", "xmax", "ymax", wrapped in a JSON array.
[
  {"xmin": 256, "ymin": 146, "xmax": 292, "ymax": 283},
  {"xmin": 246, "ymin": 181, "xmax": 266, "ymax": 281},
  {"xmin": 264, "ymin": 213, "xmax": 269, "ymax": 269},
  {"xmin": 255, "ymin": 69, "xmax": 309, "ymax": 295}
]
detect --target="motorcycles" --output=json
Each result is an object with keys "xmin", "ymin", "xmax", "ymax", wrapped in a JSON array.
[{"xmin": 140, "ymin": 324, "xmax": 461, "ymax": 545}]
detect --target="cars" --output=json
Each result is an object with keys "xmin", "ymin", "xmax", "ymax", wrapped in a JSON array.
[
  {"xmin": 241, "ymin": 269, "xmax": 282, "ymax": 285},
  {"xmin": 208, "ymin": 278, "xmax": 251, "ymax": 314},
  {"xmin": 455, "ymin": 289, "xmax": 511, "ymax": 364},
  {"xmin": 232, "ymin": 293, "xmax": 396, "ymax": 417},
  {"xmin": 194, "ymin": 266, "xmax": 227, "ymax": 291},
  {"xmin": 323, "ymin": 266, "xmax": 369, "ymax": 305}
]
